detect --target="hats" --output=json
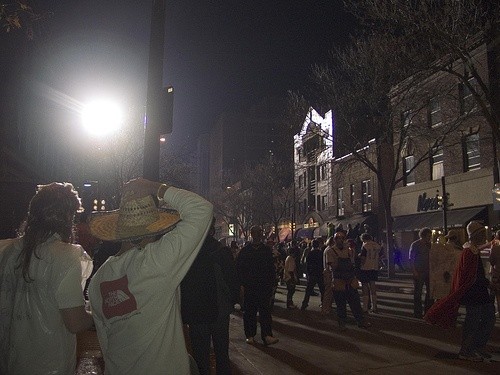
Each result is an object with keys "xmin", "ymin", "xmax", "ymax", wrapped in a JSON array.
[
  {"xmin": 444, "ymin": 230, "xmax": 457, "ymax": 239},
  {"xmin": 466, "ymin": 221, "xmax": 486, "ymax": 239},
  {"xmin": 86, "ymin": 180, "xmax": 181, "ymax": 242},
  {"xmin": 334, "ymin": 233, "xmax": 346, "ymax": 238}
]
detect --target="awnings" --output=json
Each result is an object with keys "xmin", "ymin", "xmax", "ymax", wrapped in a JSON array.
[{"xmin": 382, "ymin": 206, "xmax": 488, "ymax": 232}]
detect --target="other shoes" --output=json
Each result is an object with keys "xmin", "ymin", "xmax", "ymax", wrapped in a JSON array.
[
  {"xmin": 288, "ymin": 305, "xmax": 297, "ymax": 309},
  {"xmin": 301, "ymin": 302, "xmax": 308, "ymax": 310},
  {"xmin": 263, "ymin": 336, "xmax": 279, "ymax": 346},
  {"xmin": 245, "ymin": 336, "xmax": 255, "ymax": 344},
  {"xmin": 460, "ymin": 354, "xmax": 481, "ymax": 361}
]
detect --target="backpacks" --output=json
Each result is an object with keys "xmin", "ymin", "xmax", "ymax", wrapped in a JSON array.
[{"xmin": 331, "ymin": 246, "xmax": 353, "ymax": 280}]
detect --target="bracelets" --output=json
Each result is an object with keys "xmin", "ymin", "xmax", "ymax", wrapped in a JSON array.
[{"xmin": 153, "ymin": 182, "xmax": 166, "ymax": 200}]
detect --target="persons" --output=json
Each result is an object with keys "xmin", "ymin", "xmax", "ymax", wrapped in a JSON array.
[
  {"xmin": 87, "ymin": 178, "xmax": 213, "ymax": 375},
  {"xmin": 180, "ymin": 217, "xmax": 404, "ymax": 375},
  {"xmin": 0, "ymin": 183, "xmax": 95, "ymax": 375},
  {"xmin": 409, "ymin": 221, "xmax": 500, "ymax": 361}
]
